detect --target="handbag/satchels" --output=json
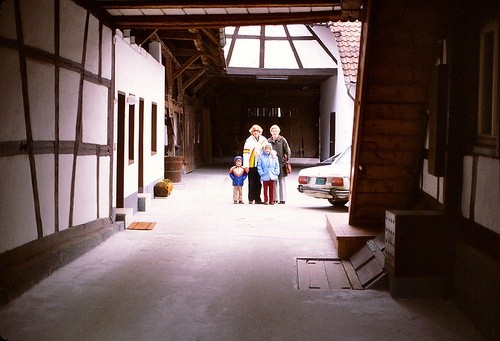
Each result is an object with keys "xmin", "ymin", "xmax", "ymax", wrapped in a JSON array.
[{"xmin": 286, "ymin": 161, "xmax": 291, "ymax": 173}]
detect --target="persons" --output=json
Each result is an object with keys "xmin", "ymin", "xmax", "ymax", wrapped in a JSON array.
[
  {"xmin": 257, "ymin": 143, "xmax": 280, "ymax": 205},
  {"xmin": 228, "ymin": 156, "xmax": 248, "ymax": 204},
  {"xmin": 267, "ymin": 125, "xmax": 291, "ymax": 204},
  {"xmin": 243, "ymin": 124, "xmax": 277, "ymax": 204}
]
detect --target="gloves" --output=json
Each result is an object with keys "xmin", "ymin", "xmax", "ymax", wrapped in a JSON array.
[{"xmin": 245, "ymin": 167, "xmax": 249, "ymax": 174}]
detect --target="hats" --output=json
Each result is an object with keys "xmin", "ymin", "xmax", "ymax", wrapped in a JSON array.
[
  {"xmin": 249, "ymin": 124, "xmax": 263, "ymax": 134},
  {"xmin": 234, "ymin": 156, "xmax": 242, "ymax": 162}
]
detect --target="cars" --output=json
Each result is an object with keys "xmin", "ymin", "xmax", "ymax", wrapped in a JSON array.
[{"xmin": 296, "ymin": 146, "xmax": 352, "ymax": 206}]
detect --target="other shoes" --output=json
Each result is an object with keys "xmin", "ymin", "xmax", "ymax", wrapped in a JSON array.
[
  {"xmin": 249, "ymin": 201, "xmax": 252, "ymax": 204},
  {"xmin": 234, "ymin": 201, "xmax": 237, "ymax": 204},
  {"xmin": 271, "ymin": 202, "xmax": 274, "ymax": 204},
  {"xmin": 265, "ymin": 202, "xmax": 269, "ymax": 205},
  {"xmin": 280, "ymin": 201, "xmax": 285, "ymax": 204},
  {"xmin": 239, "ymin": 201, "xmax": 244, "ymax": 204},
  {"xmin": 255, "ymin": 201, "xmax": 264, "ymax": 204},
  {"xmin": 274, "ymin": 201, "xmax": 278, "ymax": 204}
]
